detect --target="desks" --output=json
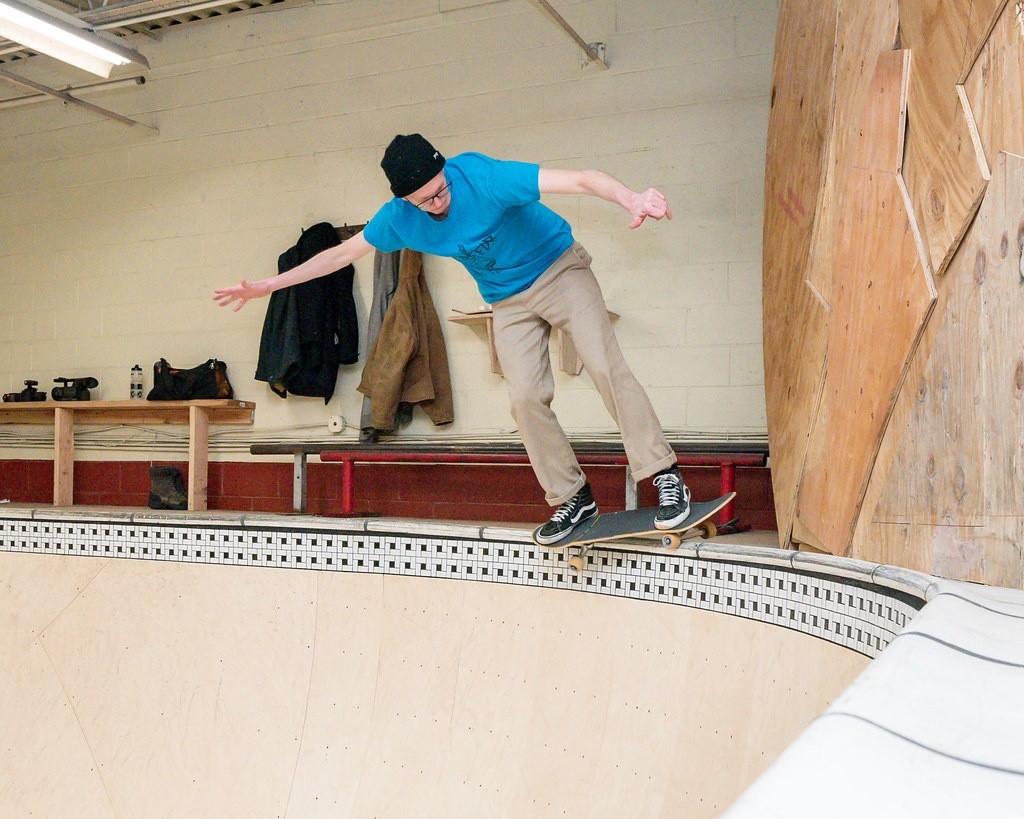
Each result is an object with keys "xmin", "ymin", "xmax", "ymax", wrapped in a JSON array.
[{"xmin": 0, "ymin": 399, "xmax": 256, "ymax": 511}]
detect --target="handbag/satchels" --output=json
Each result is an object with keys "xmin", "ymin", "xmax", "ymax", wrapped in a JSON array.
[{"xmin": 147, "ymin": 358, "xmax": 234, "ymax": 401}]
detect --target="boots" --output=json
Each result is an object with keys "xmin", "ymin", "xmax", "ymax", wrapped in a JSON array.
[
  {"xmin": 171, "ymin": 467, "xmax": 187, "ymax": 497},
  {"xmin": 148, "ymin": 466, "xmax": 188, "ymax": 510}
]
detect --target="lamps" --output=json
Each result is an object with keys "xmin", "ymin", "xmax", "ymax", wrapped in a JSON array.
[{"xmin": 0, "ymin": 0, "xmax": 151, "ymax": 78}]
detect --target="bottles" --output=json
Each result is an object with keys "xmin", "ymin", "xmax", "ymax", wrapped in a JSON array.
[{"xmin": 130, "ymin": 365, "xmax": 143, "ymax": 400}]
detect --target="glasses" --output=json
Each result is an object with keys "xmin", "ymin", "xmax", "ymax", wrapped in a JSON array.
[{"xmin": 403, "ymin": 167, "xmax": 452, "ymax": 210}]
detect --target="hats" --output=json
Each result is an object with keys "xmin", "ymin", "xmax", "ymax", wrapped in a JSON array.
[{"xmin": 380, "ymin": 134, "xmax": 446, "ymax": 198}]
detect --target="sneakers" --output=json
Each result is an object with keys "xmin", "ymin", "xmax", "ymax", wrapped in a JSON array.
[
  {"xmin": 652, "ymin": 464, "xmax": 691, "ymax": 529},
  {"xmin": 535, "ymin": 482, "xmax": 598, "ymax": 545}
]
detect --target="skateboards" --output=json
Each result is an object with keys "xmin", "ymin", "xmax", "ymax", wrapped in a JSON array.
[{"xmin": 530, "ymin": 491, "xmax": 737, "ymax": 572}]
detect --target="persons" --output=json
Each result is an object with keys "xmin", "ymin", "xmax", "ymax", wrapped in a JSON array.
[{"xmin": 212, "ymin": 133, "xmax": 691, "ymax": 546}]
